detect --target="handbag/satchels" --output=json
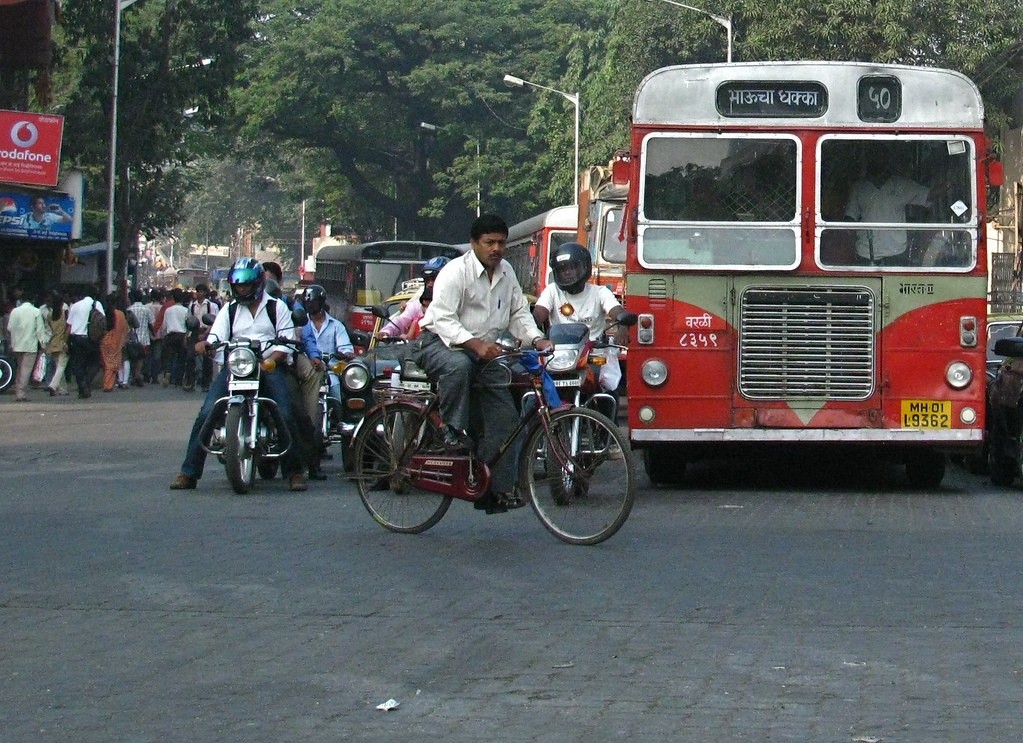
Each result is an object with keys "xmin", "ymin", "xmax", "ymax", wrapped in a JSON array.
[
  {"xmin": 126, "ymin": 310, "xmax": 140, "ymax": 328},
  {"xmin": 122, "ymin": 326, "xmax": 144, "ymax": 361},
  {"xmin": 32, "ymin": 353, "xmax": 47, "ymax": 382}
]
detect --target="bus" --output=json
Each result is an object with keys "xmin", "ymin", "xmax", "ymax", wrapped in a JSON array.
[
  {"xmin": 611, "ymin": 61, "xmax": 1005, "ymax": 490},
  {"xmin": 155, "ymin": 266, "xmax": 211, "ymax": 292},
  {"xmin": 314, "ymin": 238, "xmax": 465, "ymax": 335},
  {"xmin": 499, "ymin": 201, "xmax": 575, "ymax": 309},
  {"xmin": 584, "ymin": 196, "xmax": 629, "ymax": 311}
]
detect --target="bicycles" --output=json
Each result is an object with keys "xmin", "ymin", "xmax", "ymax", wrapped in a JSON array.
[{"xmin": 347, "ymin": 337, "xmax": 635, "ymax": 545}]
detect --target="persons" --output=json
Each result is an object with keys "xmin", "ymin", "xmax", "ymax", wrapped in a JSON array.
[
  {"xmin": 0, "ymin": 256, "xmax": 453, "ymax": 490},
  {"xmin": 843, "ymin": 152, "xmax": 955, "ymax": 266},
  {"xmin": 410, "ymin": 214, "xmax": 555, "ymax": 509},
  {"xmin": 19, "ymin": 196, "xmax": 72, "ymax": 230},
  {"xmin": 508, "ymin": 242, "xmax": 630, "ymax": 460}
]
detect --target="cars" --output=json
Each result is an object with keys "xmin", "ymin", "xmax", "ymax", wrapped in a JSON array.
[{"xmin": 949, "ymin": 315, "xmax": 1023, "ymax": 491}]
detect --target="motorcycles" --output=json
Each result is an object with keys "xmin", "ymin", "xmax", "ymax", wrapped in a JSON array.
[
  {"xmin": 520, "ymin": 312, "xmax": 638, "ymax": 507},
  {"xmin": 371, "ymin": 304, "xmax": 436, "ymax": 495},
  {"xmin": 185, "ymin": 309, "xmax": 308, "ymax": 492},
  {"xmin": 318, "ymin": 330, "xmax": 371, "ymax": 445}
]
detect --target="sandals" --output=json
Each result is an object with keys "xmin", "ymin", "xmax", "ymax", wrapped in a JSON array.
[
  {"xmin": 475, "ymin": 488, "xmax": 526, "ymax": 510},
  {"xmin": 433, "ymin": 425, "xmax": 476, "ymax": 450}
]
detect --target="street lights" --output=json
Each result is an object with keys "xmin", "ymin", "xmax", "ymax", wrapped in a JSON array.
[
  {"xmin": 504, "ymin": 75, "xmax": 579, "ymax": 207},
  {"xmin": 264, "ymin": 176, "xmax": 305, "ymax": 281},
  {"xmin": 421, "ymin": 121, "xmax": 481, "ymax": 218}
]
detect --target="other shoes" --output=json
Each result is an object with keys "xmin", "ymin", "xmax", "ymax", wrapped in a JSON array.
[
  {"xmin": 17, "ymin": 373, "xmax": 210, "ymax": 401},
  {"xmin": 290, "ymin": 472, "xmax": 308, "ymax": 490},
  {"xmin": 308, "ymin": 465, "xmax": 326, "ymax": 481},
  {"xmin": 322, "ymin": 450, "xmax": 334, "ymax": 461},
  {"xmin": 170, "ymin": 473, "xmax": 196, "ymax": 490}
]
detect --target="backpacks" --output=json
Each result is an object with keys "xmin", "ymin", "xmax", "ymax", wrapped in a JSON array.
[{"xmin": 86, "ymin": 299, "xmax": 107, "ymax": 342}]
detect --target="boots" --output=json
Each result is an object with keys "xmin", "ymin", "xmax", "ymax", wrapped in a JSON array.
[{"xmin": 598, "ymin": 390, "xmax": 620, "ymax": 444}]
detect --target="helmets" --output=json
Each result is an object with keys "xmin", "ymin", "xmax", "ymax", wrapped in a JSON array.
[
  {"xmin": 263, "ymin": 278, "xmax": 282, "ymax": 299},
  {"xmin": 420, "ymin": 255, "xmax": 453, "ymax": 279},
  {"xmin": 302, "ymin": 284, "xmax": 326, "ymax": 313},
  {"xmin": 548, "ymin": 241, "xmax": 592, "ymax": 293},
  {"xmin": 227, "ymin": 256, "xmax": 267, "ymax": 306}
]
